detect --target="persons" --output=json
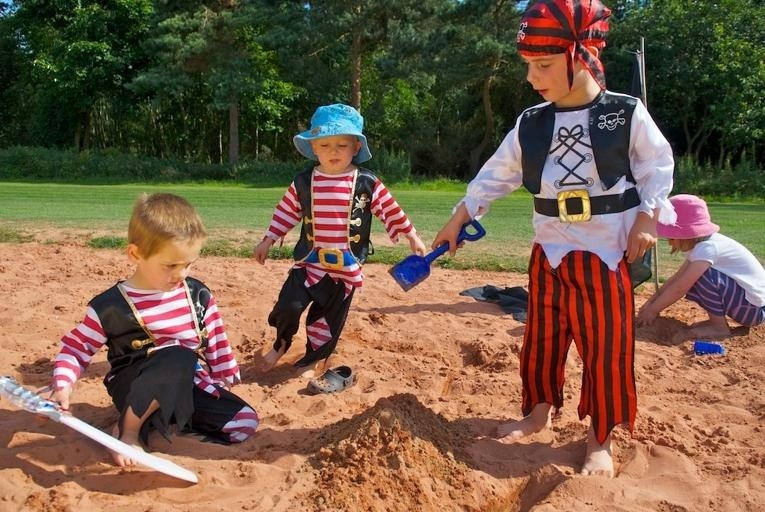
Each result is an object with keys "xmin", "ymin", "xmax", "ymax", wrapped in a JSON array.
[
  {"xmin": 46, "ymin": 191, "xmax": 259, "ymax": 467},
  {"xmin": 636, "ymin": 193, "xmax": 765, "ymax": 338},
  {"xmin": 431, "ymin": 0, "xmax": 678, "ymax": 478},
  {"xmin": 254, "ymin": 103, "xmax": 426, "ymax": 379}
]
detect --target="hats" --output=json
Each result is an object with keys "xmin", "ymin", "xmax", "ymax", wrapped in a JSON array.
[
  {"xmin": 293, "ymin": 103, "xmax": 372, "ymax": 165},
  {"xmin": 517, "ymin": 0, "xmax": 611, "ymax": 90},
  {"xmin": 656, "ymin": 194, "xmax": 720, "ymax": 240}
]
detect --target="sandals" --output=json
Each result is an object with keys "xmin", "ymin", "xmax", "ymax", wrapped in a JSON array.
[{"xmin": 308, "ymin": 365, "xmax": 353, "ymax": 394}]
